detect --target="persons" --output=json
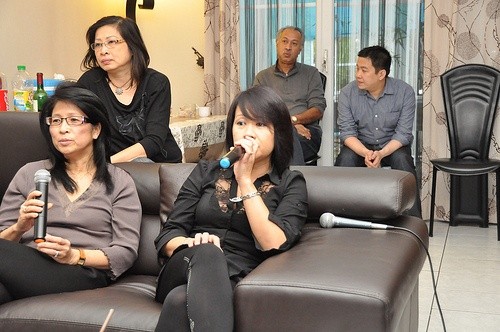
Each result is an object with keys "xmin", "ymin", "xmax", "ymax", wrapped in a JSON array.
[
  {"xmin": 252, "ymin": 26, "xmax": 327, "ymax": 162},
  {"xmin": 0, "ymin": 83, "xmax": 142, "ymax": 306},
  {"xmin": 76, "ymin": 15, "xmax": 182, "ymax": 164},
  {"xmin": 154, "ymin": 86, "xmax": 310, "ymax": 332},
  {"xmin": 333, "ymin": 46, "xmax": 422, "ymax": 219}
]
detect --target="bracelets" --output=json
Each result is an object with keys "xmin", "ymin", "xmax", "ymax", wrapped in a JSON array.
[{"xmin": 242, "ymin": 191, "xmax": 261, "ymax": 201}]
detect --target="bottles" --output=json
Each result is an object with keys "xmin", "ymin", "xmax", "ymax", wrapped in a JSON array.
[
  {"xmin": 12, "ymin": 66, "xmax": 34, "ymax": 112},
  {"xmin": 0, "ymin": 71, "xmax": 9, "ymax": 111},
  {"xmin": 33, "ymin": 73, "xmax": 47, "ymax": 111}
]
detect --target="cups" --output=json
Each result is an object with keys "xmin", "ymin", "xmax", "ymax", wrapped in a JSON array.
[{"xmin": 196, "ymin": 106, "xmax": 211, "ymax": 117}]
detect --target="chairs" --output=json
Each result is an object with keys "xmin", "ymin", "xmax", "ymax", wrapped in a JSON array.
[
  {"xmin": 305, "ymin": 71, "xmax": 327, "ymax": 166},
  {"xmin": 427, "ymin": 63, "xmax": 500, "ymax": 241}
]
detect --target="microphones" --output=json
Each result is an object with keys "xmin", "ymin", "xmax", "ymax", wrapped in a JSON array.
[
  {"xmin": 33, "ymin": 168, "xmax": 51, "ymax": 242},
  {"xmin": 319, "ymin": 212, "xmax": 395, "ymax": 230},
  {"xmin": 220, "ymin": 145, "xmax": 246, "ymax": 169}
]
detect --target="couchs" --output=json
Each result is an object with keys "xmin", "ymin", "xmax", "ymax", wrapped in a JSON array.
[{"xmin": 0, "ymin": 109, "xmax": 431, "ymax": 332}]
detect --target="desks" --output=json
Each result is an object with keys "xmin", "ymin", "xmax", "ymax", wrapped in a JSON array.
[{"xmin": 168, "ymin": 113, "xmax": 227, "ymax": 164}]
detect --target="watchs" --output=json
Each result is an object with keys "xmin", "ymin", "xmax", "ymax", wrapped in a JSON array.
[
  {"xmin": 292, "ymin": 116, "xmax": 298, "ymax": 124},
  {"xmin": 76, "ymin": 249, "xmax": 86, "ymax": 266}
]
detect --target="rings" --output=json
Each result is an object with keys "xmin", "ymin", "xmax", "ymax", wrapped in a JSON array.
[
  {"xmin": 53, "ymin": 250, "xmax": 59, "ymax": 257},
  {"xmin": 24, "ymin": 206, "xmax": 27, "ymax": 213}
]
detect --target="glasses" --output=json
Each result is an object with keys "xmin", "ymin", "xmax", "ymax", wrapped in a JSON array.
[
  {"xmin": 45, "ymin": 115, "xmax": 90, "ymax": 127},
  {"xmin": 90, "ymin": 39, "xmax": 126, "ymax": 51}
]
